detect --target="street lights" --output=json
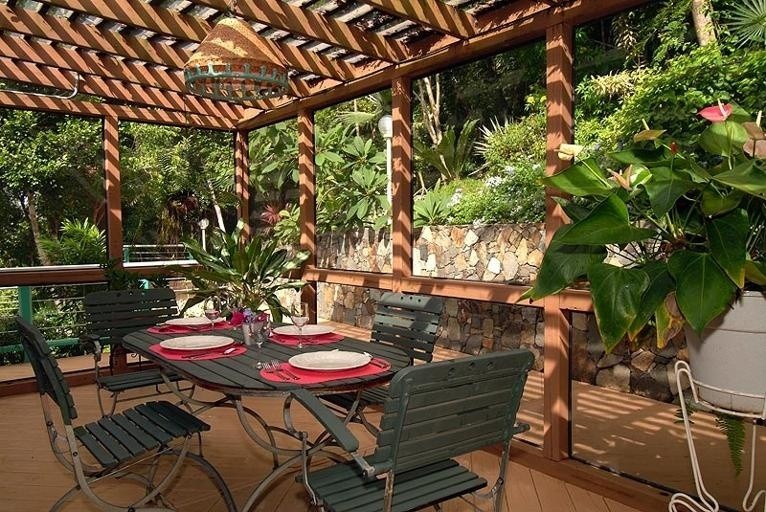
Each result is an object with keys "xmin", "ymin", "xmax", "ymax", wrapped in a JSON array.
[
  {"xmin": 377, "ymin": 114, "xmax": 394, "ymax": 226},
  {"xmin": 198, "ymin": 219, "xmax": 210, "ymax": 252}
]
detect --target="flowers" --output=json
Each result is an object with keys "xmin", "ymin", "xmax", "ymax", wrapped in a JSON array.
[{"xmin": 512, "ymin": 97, "xmax": 766, "ymax": 357}]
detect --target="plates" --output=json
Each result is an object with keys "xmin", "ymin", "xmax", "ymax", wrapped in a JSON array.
[
  {"xmin": 164, "ymin": 316, "xmax": 225, "ymax": 327},
  {"xmin": 159, "ymin": 335, "xmax": 235, "ymax": 352},
  {"xmin": 272, "ymin": 324, "xmax": 335, "ymax": 336},
  {"xmin": 288, "ymin": 349, "xmax": 372, "ymax": 371}
]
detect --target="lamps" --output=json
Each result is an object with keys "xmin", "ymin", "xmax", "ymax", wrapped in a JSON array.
[{"xmin": 182, "ymin": 1, "xmax": 288, "ymax": 102}]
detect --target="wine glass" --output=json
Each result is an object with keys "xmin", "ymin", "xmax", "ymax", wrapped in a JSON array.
[
  {"xmin": 203, "ymin": 295, "xmax": 223, "ymax": 331},
  {"xmin": 241, "ymin": 314, "xmax": 273, "ymax": 368},
  {"xmin": 289, "ymin": 300, "xmax": 311, "ymax": 349}
]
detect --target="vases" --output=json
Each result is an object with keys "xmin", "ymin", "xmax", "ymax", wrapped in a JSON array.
[{"xmin": 683, "ymin": 290, "xmax": 766, "ymax": 416}]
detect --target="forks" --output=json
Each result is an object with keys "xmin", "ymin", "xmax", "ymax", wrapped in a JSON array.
[
  {"xmin": 270, "ymin": 359, "xmax": 300, "ymax": 380},
  {"xmin": 182, "ymin": 347, "xmax": 240, "ymax": 359},
  {"xmin": 260, "ymin": 359, "xmax": 290, "ymax": 381}
]
